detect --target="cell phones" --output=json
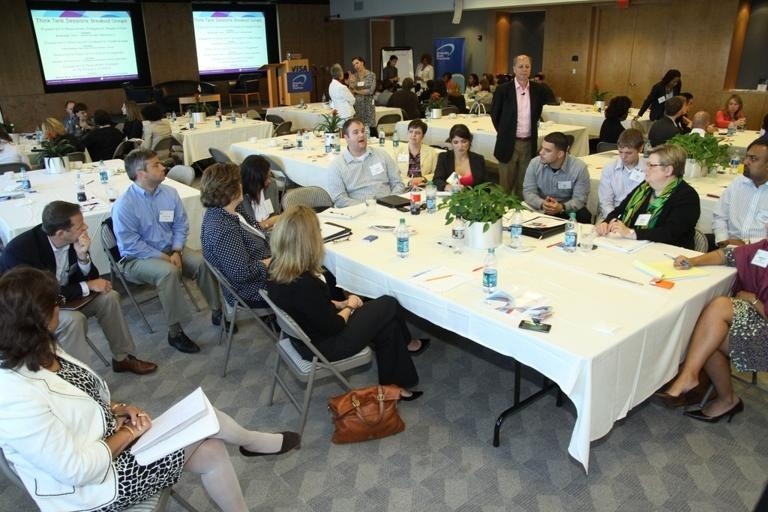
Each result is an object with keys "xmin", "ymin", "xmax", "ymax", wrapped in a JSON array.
[{"xmin": 518, "ymin": 319, "xmax": 552, "ymax": 333}]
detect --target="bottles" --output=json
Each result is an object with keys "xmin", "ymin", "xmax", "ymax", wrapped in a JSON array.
[
  {"xmin": 730, "ymin": 150, "xmax": 740, "ymax": 175},
  {"xmin": 395, "ymin": 172, "xmax": 578, "ymax": 296},
  {"xmin": 321, "ymin": 93, "xmax": 326, "ymax": 106},
  {"xmin": 300, "ymin": 97, "xmax": 305, "ymax": 111},
  {"xmin": 171, "ymin": 107, "xmax": 237, "ymax": 131},
  {"xmin": 20, "ymin": 165, "xmax": 31, "ymax": 195},
  {"xmin": 283, "ymin": 125, "xmax": 399, "ymax": 154},
  {"xmin": 425, "ymin": 106, "xmax": 432, "ymax": 125},
  {"xmin": 76, "ymin": 160, "xmax": 111, "ymax": 202},
  {"xmin": 727, "ymin": 119, "xmax": 735, "ymax": 139}
]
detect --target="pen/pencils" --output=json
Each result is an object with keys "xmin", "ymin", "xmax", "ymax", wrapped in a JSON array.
[
  {"xmin": 413, "ymin": 269, "xmax": 432, "ymax": 277},
  {"xmin": 707, "ymin": 194, "xmax": 720, "ymax": 199},
  {"xmin": 472, "ymin": 265, "xmax": 488, "ymax": 272},
  {"xmin": 610, "ymin": 214, "xmax": 621, "ymax": 232},
  {"xmin": 426, "ymin": 275, "xmax": 452, "ymax": 281},
  {"xmin": 83, "ymin": 202, "xmax": 99, "ymax": 211},
  {"xmin": 664, "ymin": 254, "xmax": 688, "ymax": 266},
  {"xmin": 111, "ymin": 414, "xmax": 145, "ymax": 417},
  {"xmin": 87, "ymin": 180, "xmax": 94, "ymax": 185},
  {"xmin": 330, "ymin": 212, "xmax": 344, "ymax": 215}
]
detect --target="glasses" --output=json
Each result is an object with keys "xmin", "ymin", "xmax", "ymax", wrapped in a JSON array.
[{"xmin": 646, "ymin": 161, "xmax": 665, "ymax": 169}]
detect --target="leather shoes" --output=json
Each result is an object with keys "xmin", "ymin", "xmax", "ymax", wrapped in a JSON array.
[
  {"xmin": 408, "ymin": 338, "xmax": 431, "ymax": 357},
  {"xmin": 167, "ymin": 330, "xmax": 200, "ymax": 354},
  {"xmin": 400, "ymin": 390, "xmax": 424, "ymax": 401},
  {"xmin": 212, "ymin": 314, "xmax": 240, "ymax": 333},
  {"xmin": 111, "ymin": 354, "xmax": 157, "ymax": 374}
]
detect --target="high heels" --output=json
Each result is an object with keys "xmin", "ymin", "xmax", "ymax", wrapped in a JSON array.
[
  {"xmin": 649, "ymin": 384, "xmax": 701, "ymax": 407},
  {"xmin": 684, "ymin": 397, "xmax": 744, "ymax": 423}
]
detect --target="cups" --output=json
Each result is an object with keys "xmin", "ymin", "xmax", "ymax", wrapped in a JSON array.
[
  {"xmin": 166, "ymin": 113, "xmax": 171, "ymax": 120},
  {"xmin": 17, "ymin": 129, "xmax": 43, "ymax": 153},
  {"xmin": 242, "ymin": 112, "xmax": 247, "ymax": 124},
  {"xmin": 581, "ymin": 225, "xmax": 594, "ymax": 254},
  {"xmin": 737, "ymin": 163, "xmax": 745, "ymax": 174},
  {"xmin": 365, "ymin": 193, "xmax": 377, "ymax": 219},
  {"xmin": 108, "ymin": 187, "xmax": 116, "ymax": 202},
  {"xmin": 311, "ymin": 148, "xmax": 318, "ymax": 162},
  {"xmin": 740, "ymin": 121, "xmax": 745, "ymax": 133},
  {"xmin": 248, "ymin": 136, "xmax": 257, "ymax": 144},
  {"xmin": 3, "ymin": 170, "xmax": 17, "ymax": 191}
]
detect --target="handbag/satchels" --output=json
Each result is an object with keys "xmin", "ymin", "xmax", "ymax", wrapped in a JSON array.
[{"xmin": 327, "ymin": 384, "xmax": 406, "ymax": 444}]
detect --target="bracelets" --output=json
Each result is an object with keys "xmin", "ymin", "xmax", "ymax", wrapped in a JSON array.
[
  {"xmin": 119, "ymin": 425, "xmax": 135, "ymax": 441},
  {"xmin": 750, "ymin": 298, "xmax": 760, "ymax": 307}
]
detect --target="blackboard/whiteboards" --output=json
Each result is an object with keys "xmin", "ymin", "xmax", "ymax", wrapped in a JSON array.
[{"xmin": 381, "ymin": 46, "xmax": 415, "ymax": 86}]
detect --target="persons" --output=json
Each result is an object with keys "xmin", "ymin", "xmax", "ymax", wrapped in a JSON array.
[
  {"xmin": 1, "ymin": 267, "xmax": 301, "ymax": 512},
  {"xmin": 61, "ymin": 98, "xmax": 174, "ymax": 161},
  {"xmin": 490, "ymin": 55, "xmax": 543, "ymax": 195},
  {"xmin": 373, "ymin": 47, "xmax": 514, "ymax": 118},
  {"xmin": 1, "ymin": 200, "xmax": 156, "ymax": 376},
  {"xmin": 522, "ymin": 131, "xmax": 592, "ymax": 224},
  {"xmin": 715, "ymin": 94, "xmax": 747, "ymax": 129},
  {"xmin": 239, "ymin": 154, "xmax": 282, "ymax": 232},
  {"xmin": 653, "ymin": 224, "xmax": 768, "ymax": 424},
  {"xmin": 329, "ymin": 57, "xmax": 380, "ymax": 137},
  {"xmin": 110, "ymin": 149, "xmax": 238, "ymax": 354},
  {"xmin": 200, "ymin": 160, "xmax": 346, "ymax": 337},
  {"xmin": 713, "ymin": 135, "xmax": 768, "ymax": 250},
  {"xmin": 595, "ymin": 127, "xmax": 701, "ymax": 252},
  {"xmin": 599, "ymin": 69, "xmax": 701, "ymax": 150},
  {"xmin": 324, "ymin": 118, "xmax": 486, "ymax": 208},
  {"xmin": 529, "ymin": 72, "xmax": 557, "ymax": 104},
  {"xmin": 266, "ymin": 206, "xmax": 431, "ymax": 401}
]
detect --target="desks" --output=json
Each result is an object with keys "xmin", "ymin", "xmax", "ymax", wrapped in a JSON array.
[{"xmin": 1, "ymin": 93, "xmax": 768, "ymax": 449}]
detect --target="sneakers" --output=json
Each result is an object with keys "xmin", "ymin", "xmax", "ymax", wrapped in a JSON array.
[{"xmin": 240, "ymin": 431, "xmax": 301, "ymax": 457}]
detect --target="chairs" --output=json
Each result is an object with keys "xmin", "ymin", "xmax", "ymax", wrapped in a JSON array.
[
  {"xmin": 121, "ymin": 73, "xmax": 261, "ymax": 118},
  {"xmin": 0, "ymin": 444, "xmax": 197, "ymax": 511}
]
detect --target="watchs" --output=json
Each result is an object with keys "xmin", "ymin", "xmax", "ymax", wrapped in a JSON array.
[
  {"xmin": 347, "ymin": 306, "xmax": 355, "ymax": 316},
  {"xmin": 111, "ymin": 402, "xmax": 127, "ymax": 413}
]
